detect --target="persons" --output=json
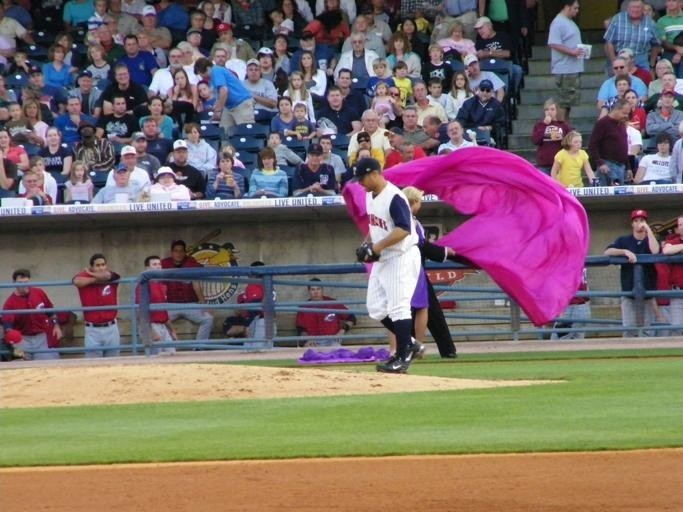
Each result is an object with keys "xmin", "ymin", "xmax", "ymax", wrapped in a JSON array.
[
  {"xmin": 349, "ymin": 157, "xmax": 426, "ymax": 374},
  {"xmin": 387, "ymin": 219, "xmax": 429, "ymax": 361},
  {"xmin": 603, "ymin": 209, "xmax": 683, "ymax": 336},
  {"xmin": 161, "ymin": 239, "xmax": 214, "ymax": 351},
  {"xmin": 222, "ymin": 261, "xmax": 277, "ymax": 349},
  {"xmin": 538, "ymin": 270, "xmax": 591, "ymax": 340},
  {"xmin": 0, "ymin": 269, "xmax": 63, "ymax": 360},
  {"xmin": 295, "ymin": 277, "xmax": 357, "ymax": 347},
  {"xmin": 0, "ymin": 1, "xmax": 682, "ymax": 204},
  {"xmin": 45, "ymin": 312, "xmax": 77, "ymax": 359},
  {"xmin": 72, "ymin": 253, "xmax": 120, "ymax": 358},
  {"xmin": 135, "ymin": 256, "xmax": 178, "ymax": 353},
  {"xmin": 401, "ymin": 186, "xmax": 460, "ymax": 361}
]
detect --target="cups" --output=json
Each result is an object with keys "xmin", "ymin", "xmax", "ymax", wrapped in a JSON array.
[
  {"xmin": 592, "ymin": 177, "xmax": 600, "ymax": 187},
  {"xmin": 585, "ymin": 45, "xmax": 592, "ymax": 59},
  {"xmin": 576, "ymin": 44, "xmax": 585, "ymax": 59},
  {"xmin": 225, "ymin": 175, "xmax": 232, "ymax": 186},
  {"xmin": 25, "ymin": 199, "xmax": 33, "ymax": 207}
]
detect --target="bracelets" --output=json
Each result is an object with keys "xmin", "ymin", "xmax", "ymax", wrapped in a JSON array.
[
  {"xmin": 51, "ymin": 318, "xmax": 59, "ymax": 324},
  {"xmin": 341, "ymin": 322, "xmax": 350, "ymax": 334}
]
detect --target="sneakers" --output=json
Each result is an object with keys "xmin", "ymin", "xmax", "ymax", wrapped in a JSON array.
[
  {"xmin": 405, "ymin": 336, "xmax": 424, "ymax": 363},
  {"xmin": 377, "ymin": 356, "xmax": 407, "ymax": 374}
]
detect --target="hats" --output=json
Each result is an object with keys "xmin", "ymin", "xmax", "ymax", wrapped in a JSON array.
[
  {"xmin": 247, "ymin": 46, "xmax": 273, "ymax": 67},
  {"xmin": 662, "ymin": 87, "xmax": 675, "ymax": 95},
  {"xmin": 238, "ymin": 284, "xmax": 263, "ymax": 303},
  {"xmin": 78, "ymin": 69, "xmax": 92, "ymax": 78},
  {"xmin": 350, "ymin": 158, "xmax": 380, "ymax": 182},
  {"xmin": 464, "ymin": 55, "xmax": 493, "ymax": 89},
  {"xmin": 631, "ymin": 210, "xmax": 648, "ymax": 219},
  {"xmin": 308, "ymin": 143, "xmax": 323, "ymax": 154},
  {"xmin": 473, "ymin": 16, "xmax": 491, "ymax": 28},
  {"xmin": 28, "ymin": 66, "xmax": 41, "ymax": 76},
  {"xmin": 113, "ymin": 132, "xmax": 188, "ymax": 178},
  {"xmin": 143, "ymin": 5, "xmax": 156, "ymax": 17},
  {"xmin": 78, "ymin": 120, "xmax": 96, "ymax": 133}
]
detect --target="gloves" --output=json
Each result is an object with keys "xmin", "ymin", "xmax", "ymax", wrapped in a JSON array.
[
  {"xmin": 356, "ymin": 243, "xmax": 379, "ymax": 262},
  {"xmin": 4, "ymin": 329, "xmax": 21, "ymax": 343}
]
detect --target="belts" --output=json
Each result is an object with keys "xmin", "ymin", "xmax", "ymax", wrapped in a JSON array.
[
  {"xmin": 86, "ymin": 321, "xmax": 114, "ymax": 327},
  {"xmin": 673, "ymin": 286, "xmax": 683, "ymax": 290}
]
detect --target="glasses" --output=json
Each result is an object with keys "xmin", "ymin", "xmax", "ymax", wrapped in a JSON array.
[
  {"xmin": 614, "ymin": 66, "xmax": 624, "ymax": 69},
  {"xmin": 481, "ymin": 87, "xmax": 491, "ymax": 92}
]
